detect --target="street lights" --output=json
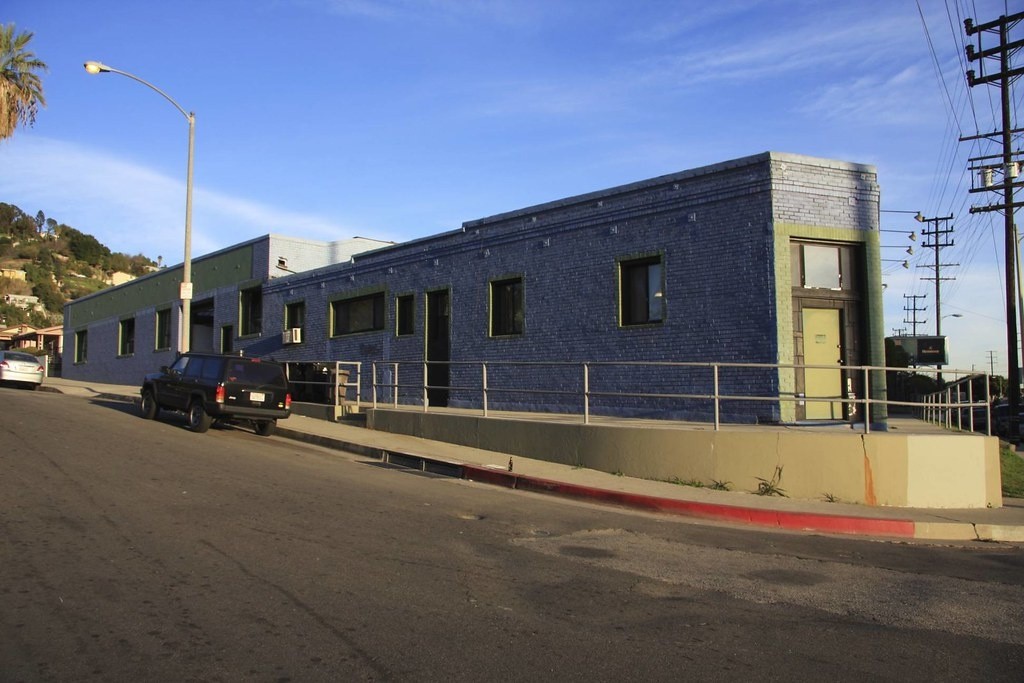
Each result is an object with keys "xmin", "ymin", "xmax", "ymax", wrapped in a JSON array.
[
  {"xmin": 935, "ymin": 314, "xmax": 964, "ymax": 380},
  {"xmin": 83, "ymin": 61, "xmax": 198, "ymax": 357}
]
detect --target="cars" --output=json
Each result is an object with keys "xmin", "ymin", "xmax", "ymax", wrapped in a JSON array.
[{"xmin": 0, "ymin": 350, "xmax": 45, "ymax": 391}]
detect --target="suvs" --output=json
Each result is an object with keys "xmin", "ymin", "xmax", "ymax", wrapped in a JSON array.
[{"xmin": 140, "ymin": 349, "xmax": 294, "ymax": 438}]
